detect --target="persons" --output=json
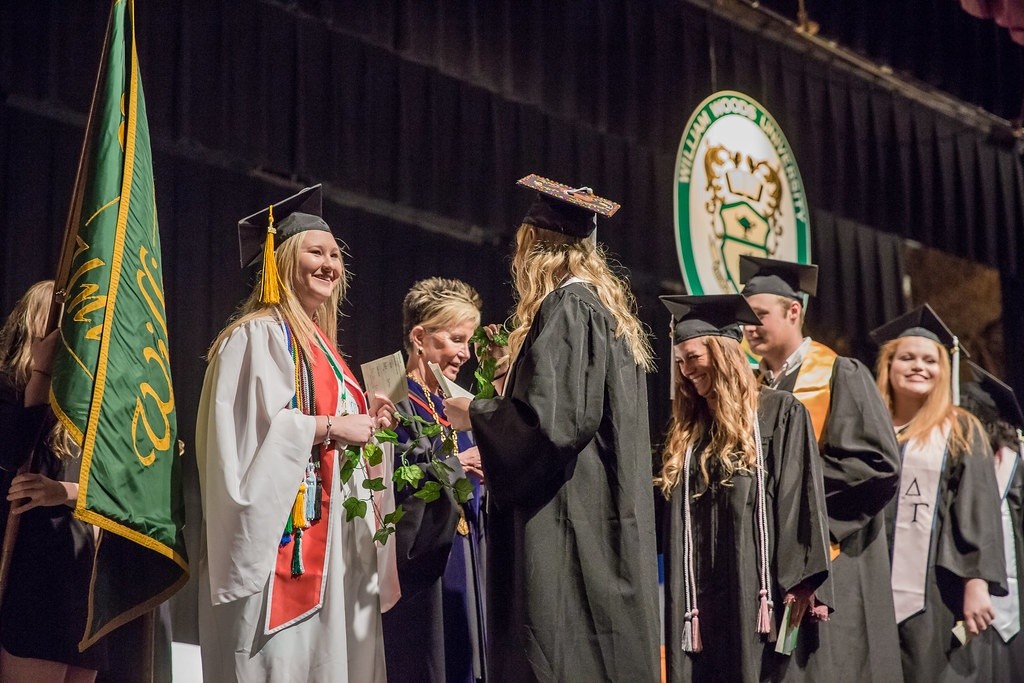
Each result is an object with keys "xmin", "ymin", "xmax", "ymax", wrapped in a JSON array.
[
  {"xmin": 659, "ymin": 292, "xmax": 835, "ymax": 683},
  {"xmin": 0, "ymin": 281, "xmax": 110, "ymax": 683},
  {"xmin": 440, "ymin": 173, "xmax": 660, "ymax": 683},
  {"xmin": 382, "ymin": 278, "xmax": 489, "ymax": 683},
  {"xmin": 193, "ymin": 183, "xmax": 401, "ymax": 683},
  {"xmin": 958, "ymin": 359, "xmax": 1024, "ymax": 683},
  {"xmin": 734, "ymin": 255, "xmax": 904, "ymax": 682},
  {"xmin": 869, "ymin": 302, "xmax": 1009, "ymax": 683}
]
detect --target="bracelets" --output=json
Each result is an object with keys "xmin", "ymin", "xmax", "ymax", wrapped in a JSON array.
[
  {"xmin": 490, "ymin": 368, "xmax": 509, "ymax": 382},
  {"xmin": 322, "ymin": 414, "xmax": 333, "ymax": 449}
]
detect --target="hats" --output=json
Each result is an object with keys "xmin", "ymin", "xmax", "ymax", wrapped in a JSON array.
[
  {"xmin": 740, "ymin": 255, "xmax": 818, "ymax": 310},
  {"xmin": 658, "ymin": 294, "xmax": 765, "ymax": 401},
  {"xmin": 964, "ymin": 360, "xmax": 1024, "ymax": 432},
  {"xmin": 517, "ymin": 174, "xmax": 621, "ymax": 239},
  {"xmin": 239, "ymin": 183, "xmax": 330, "ymax": 303},
  {"xmin": 868, "ymin": 303, "xmax": 970, "ymax": 405}
]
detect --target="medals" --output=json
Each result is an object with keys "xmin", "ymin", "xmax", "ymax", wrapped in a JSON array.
[{"xmin": 457, "ymin": 510, "xmax": 469, "ymax": 536}]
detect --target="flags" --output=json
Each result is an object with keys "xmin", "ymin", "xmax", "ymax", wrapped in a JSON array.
[{"xmin": 50, "ymin": 0, "xmax": 192, "ymax": 650}]
